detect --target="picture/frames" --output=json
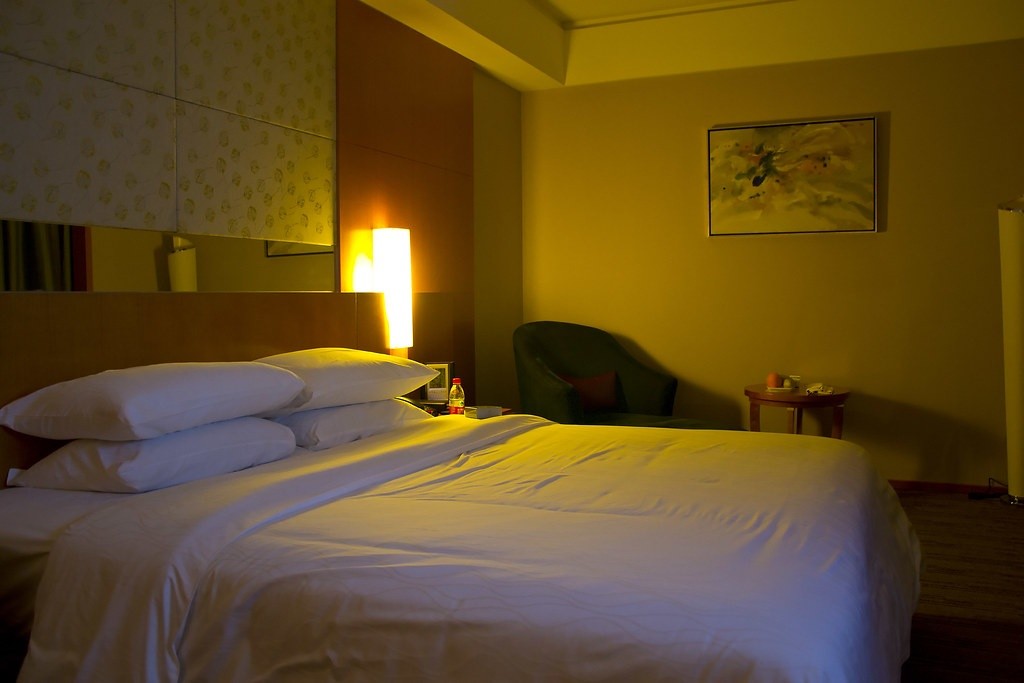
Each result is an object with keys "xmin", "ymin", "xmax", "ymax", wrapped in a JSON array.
[
  {"xmin": 264, "ymin": 240, "xmax": 335, "ymax": 257},
  {"xmin": 705, "ymin": 115, "xmax": 878, "ymax": 238}
]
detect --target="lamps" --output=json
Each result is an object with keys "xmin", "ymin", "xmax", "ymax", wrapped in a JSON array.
[{"xmin": 373, "ymin": 227, "xmax": 414, "ymax": 348}]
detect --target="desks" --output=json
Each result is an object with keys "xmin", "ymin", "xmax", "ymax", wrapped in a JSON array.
[{"xmin": 743, "ymin": 383, "xmax": 851, "ymax": 439}]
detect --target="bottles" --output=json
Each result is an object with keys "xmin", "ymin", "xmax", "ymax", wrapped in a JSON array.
[{"xmin": 449, "ymin": 377, "xmax": 465, "ymax": 415}]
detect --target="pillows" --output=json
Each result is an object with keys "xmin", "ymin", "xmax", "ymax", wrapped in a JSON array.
[
  {"xmin": 254, "ymin": 347, "xmax": 441, "ymax": 414},
  {"xmin": 560, "ymin": 369, "xmax": 621, "ymax": 413},
  {"xmin": 272, "ymin": 399, "xmax": 435, "ymax": 452},
  {"xmin": 6, "ymin": 417, "xmax": 297, "ymax": 493},
  {"xmin": 0, "ymin": 361, "xmax": 306, "ymax": 443}
]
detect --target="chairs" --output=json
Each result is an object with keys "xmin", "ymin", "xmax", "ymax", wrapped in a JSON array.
[{"xmin": 512, "ymin": 320, "xmax": 679, "ymax": 427}]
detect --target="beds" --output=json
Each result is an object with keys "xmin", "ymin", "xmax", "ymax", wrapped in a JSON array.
[{"xmin": 0, "ymin": 292, "xmax": 923, "ymax": 683}]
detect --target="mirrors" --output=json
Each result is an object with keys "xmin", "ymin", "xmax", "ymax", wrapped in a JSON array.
[{"xmin": 0, "ymin": 218, "xmax": 337, "ymax": 292}]
